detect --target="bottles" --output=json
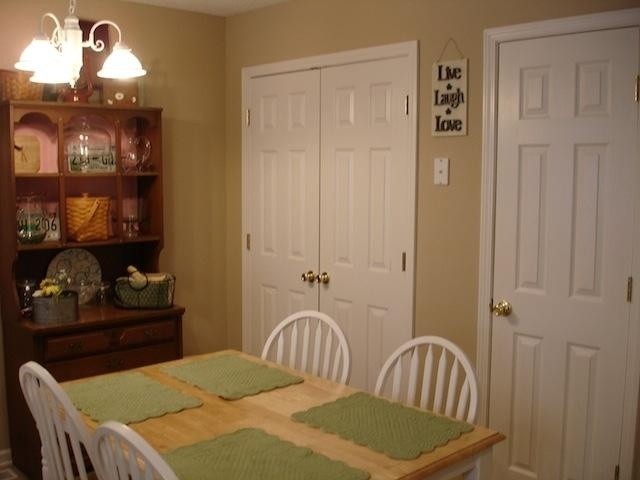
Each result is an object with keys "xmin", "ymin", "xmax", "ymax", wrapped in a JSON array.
[
  {"xmin": 123, "ymin": 214, "xmax": 139, "ymax": 239},
  {"xmin": 15, "ymin": 192, "xmax": 49, "ymax": 245}
]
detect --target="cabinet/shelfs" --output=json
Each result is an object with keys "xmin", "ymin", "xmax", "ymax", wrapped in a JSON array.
[{"xmin": 0, "ymin": 97, "xmax": 185, "ymax": 479}]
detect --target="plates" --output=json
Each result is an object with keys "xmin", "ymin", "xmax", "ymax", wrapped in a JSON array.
[{"xmin": 45, "ymin": 247, "xmax": 104, "ymax": 308}]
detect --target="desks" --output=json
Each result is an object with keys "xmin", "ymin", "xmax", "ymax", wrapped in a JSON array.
[{"xmin": 38, "ymin": 348, "xmax": 508, "ymax": 479}]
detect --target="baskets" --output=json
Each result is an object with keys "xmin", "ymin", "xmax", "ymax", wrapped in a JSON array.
[
  {"xmin": 62, "ymin": 191, "xmax": 111, "ymax": 242},
  {"xmin": 111, "ymin": 265, "xmax": 176, "ymax": 310}
]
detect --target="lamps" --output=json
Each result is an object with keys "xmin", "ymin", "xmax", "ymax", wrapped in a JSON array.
[{"xmin": 13, "ymin": 1, "xmax": 147, "ymax": 87}]
